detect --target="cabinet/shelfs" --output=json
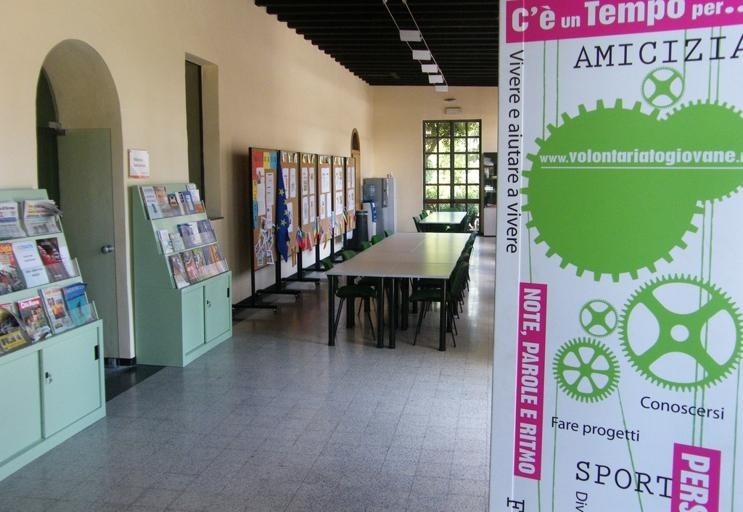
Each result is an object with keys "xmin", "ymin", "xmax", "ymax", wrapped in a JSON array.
[
  {"xmin": 132, "ymin": 183, "xmax": 232, "ymax": 367},
  {"xmin": 0, "ymin": 188, "xmax": 107, "ymax": 484},
  {"xmin": 481, "ymin": 152, "xmax": 497, "ymax": 237}
]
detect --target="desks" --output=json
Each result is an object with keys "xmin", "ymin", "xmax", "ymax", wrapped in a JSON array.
[
  {"xmin": 419, "ymin": 211, "xmax": 468, "ymax": 232},
  {"xmin": 325, "ymin": 232, "xmax": 472, "ymax": 351}
]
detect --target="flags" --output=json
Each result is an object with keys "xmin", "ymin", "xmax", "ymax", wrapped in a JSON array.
[
  {"xmin": 275, "ymin": 160, "xmax": 291, "ymax": 262},
  {"xmin": 296, "ymin": 208, "xmax": 352, "ymax": 252}
]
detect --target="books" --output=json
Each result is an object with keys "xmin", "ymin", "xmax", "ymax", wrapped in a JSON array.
[
  {"xmin": 141, "ymin": 181, "xmax": 228, "ymax": 289},
  {"xmin": 1, "ymin": 199, "xmax": 95, "ymax": 357}
]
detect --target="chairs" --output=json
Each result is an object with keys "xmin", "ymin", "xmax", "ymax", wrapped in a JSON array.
[
  {"xmin": 361, "ymin": 230, "xmax": 392, "ymax": 249},
  {"xmin": 423, "ymin": 252, "xmax": 469, "ymax": 319},
  {"xmin": 319, "ymin": 258, "xmax": 376, "ymax": 341},
  {"xmin": 409, "ymin": 261, "xmax": 469, "ymax": 347},
  {"xmin": 413, "ymin": 217, "xmax": 421, "ymax": 232},
  {"xmin": 459, "ymin": 232, "xmax": 478, "ymax": 291},
  {"xmin": 419, "ymin": 211, "xmax": 428, "ymax": 220},
  {"xmin": 341, "ymin": 250, "xmax": 390, "ymax": 316}
]
